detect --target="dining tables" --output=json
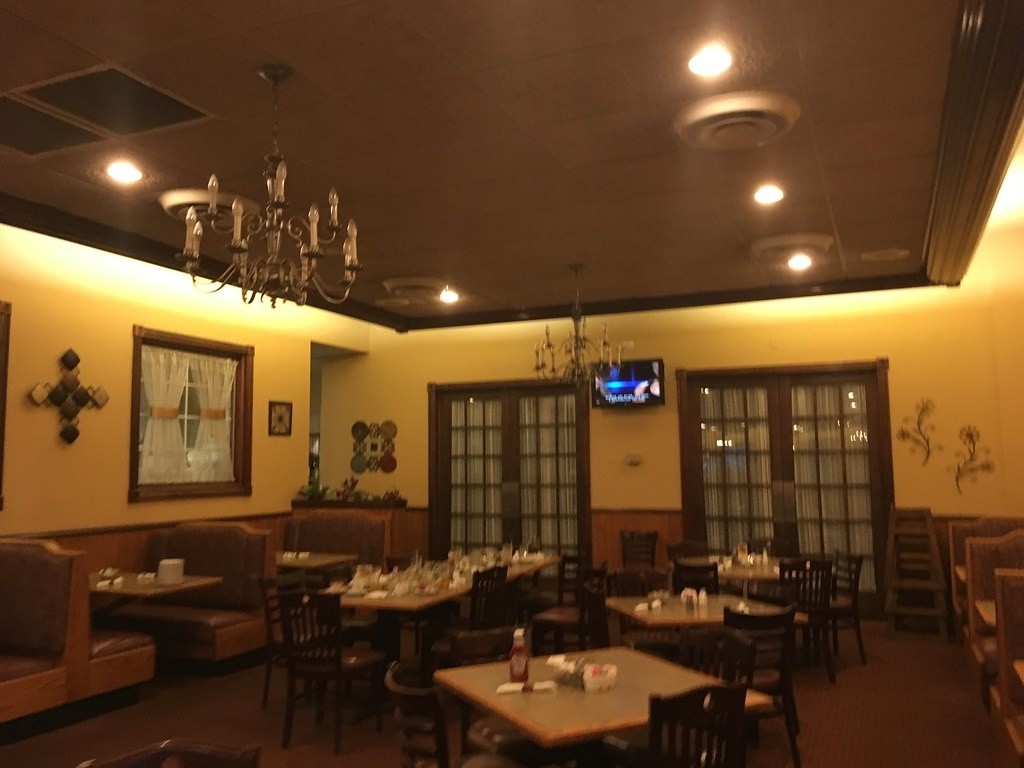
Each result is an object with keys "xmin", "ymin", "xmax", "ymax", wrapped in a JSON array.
[
  {"xmin": 432, "ymin": 644, "xmax": 776, "ymax": 766},
  {"xmin": 677, "ymin": 552, "xmax": 813, "ymax": 606},
  {"xmin": 328, "ymin": 555, "xmax": 564, "ymax": 685},
  {"xmin": 84, "ymin": 567, "xmax": 223, "ymax": 601},
  {"xmin": 601, "ymin": 592, "xmax": 794, "ymax": 682},
  {"xmin": 274, "ymin": 547, "xmax": 354, "ymax": 568}
]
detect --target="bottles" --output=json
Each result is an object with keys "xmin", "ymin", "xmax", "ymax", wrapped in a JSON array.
[{"xmin": 509, "ymin": 628, "xmax": 528, "ymax": 682}]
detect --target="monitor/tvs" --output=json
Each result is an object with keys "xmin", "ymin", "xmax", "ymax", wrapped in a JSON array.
[{"xmin": 590, "ymin": 357, "xmax": 664, "ymax": 408}]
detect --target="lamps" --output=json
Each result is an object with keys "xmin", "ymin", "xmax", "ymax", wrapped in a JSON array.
[
  {"xmin": 533, "ymin": 263, "xmax": 623, "ymax": 390},
  {"xmin": 159, "ymin": 52, "xmax": 362, "ymax": 305}
]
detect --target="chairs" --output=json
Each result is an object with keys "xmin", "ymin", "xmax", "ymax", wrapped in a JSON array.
[
  {"xmin": 633, "ymin": 680, "xmax": 751, "ymax": 766},
  {"xmin": 449, "ymin": 565, "xmax": 509, "ymax": 634},
  {"xmin": 671, "ymin": 558, "xmax": 722, "ymax": 595},
  {"xmin": 378, "ymin": 657, "xmax": 512, "ymax": 768},
  {"xmin": 256, "ymin": 577, "xmax": 328, "ymax": 712},
  {"xmin": 618, "ymin": 529, "xmax": 658, "ymax": 573},
  {"xmin": 826, "ymin": 550, "xmax": 869, "ymax": 663},
  {"xmin": 715, "ymin": 606, "xmax": 805, "ymax": 738},
  {"xmin": 680, "ymin": 627, "xmax": 759, "ymax": 692},
  {"xmin": 279, "ymin": 590, "xmax": 386, "ymax": 754},
  {"xmin": 773, "ymin": 560, "xmax": 838, "ymax": 684},
  {"xmin": 531, "ymin": 567, "xmax": 608, "ymax": 655}
]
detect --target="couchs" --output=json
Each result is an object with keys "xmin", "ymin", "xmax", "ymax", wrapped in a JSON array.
[
  {"xmin": 140, "ymin": 526, "xmax": 270, "ymax": 668},
  {"xmin": 944, "ymin": 516, "xmax": 1024, "ymax": 613},
  {"xmin": 80, "ymin": 554, "xmax": 158, "ymax": 708},
  {"xmin": 281, "ymin": 508, "xmax": 390, "ymax": 578},
  {"xmin": 988, "ymin": 568, "xmax": 1023, "ymax": 734},
  {"xmin": 2, "ymin": 540, "xmax": 76, "ymax": 737},
  {"xmin": 966, "ymin": 530, "xmax": 1024, "ymax": 674}
]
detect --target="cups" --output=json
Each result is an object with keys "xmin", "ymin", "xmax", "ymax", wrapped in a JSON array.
[
  {"xmin": 723, "ymin": 557, "xmax": 733, "ymax": 567},
  {"xmin": 709, "ymin": 556, "xmax": 719, "ymax": 565},
  {"xmin": 369, "ymin": 566, "xmax": 381, "ymax": 590}
]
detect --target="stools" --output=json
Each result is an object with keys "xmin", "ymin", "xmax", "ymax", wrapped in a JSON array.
[{"xmin": 884, "ymin": 505, "xmax": 952, "ymax": 642}]
